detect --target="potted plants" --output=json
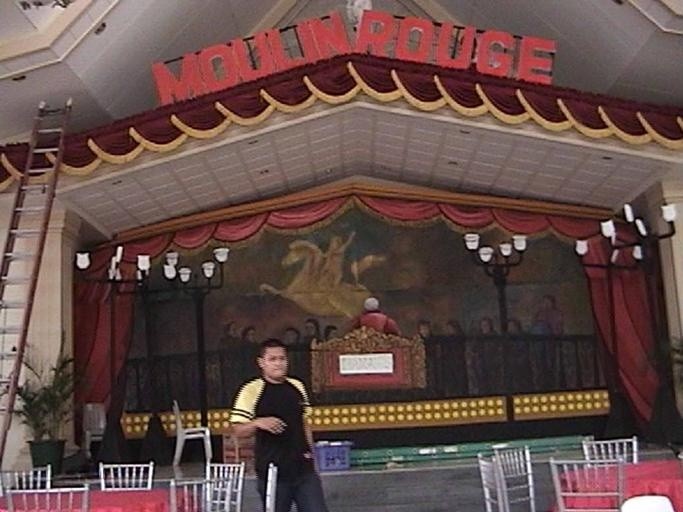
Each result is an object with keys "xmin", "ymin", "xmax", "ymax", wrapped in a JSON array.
[{"xmin": 0, "ymin": 332, "xmax": 85, "ymax": 474}]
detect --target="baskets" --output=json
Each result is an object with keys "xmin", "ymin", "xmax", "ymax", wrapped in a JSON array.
[{"xmin": 314, "ymin": 442, "xmax": 352, "ymax": 471}]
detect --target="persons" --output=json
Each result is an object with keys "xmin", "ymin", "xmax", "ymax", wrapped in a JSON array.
[{"xmin": 228, "ymin": 338, "xmax": 327, "ymax": 512}]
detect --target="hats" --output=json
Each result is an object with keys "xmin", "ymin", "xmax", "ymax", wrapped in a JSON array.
[{"xmin": 364, "ymin": 297, "xmax": 378, "ymax": 311}]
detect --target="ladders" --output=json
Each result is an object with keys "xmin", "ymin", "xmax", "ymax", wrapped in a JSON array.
[{"xmin": 1, "ymin": 98, "xmax": 72, "ymax": 467}]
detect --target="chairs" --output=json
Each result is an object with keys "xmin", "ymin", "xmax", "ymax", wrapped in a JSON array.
[
  {"xmin": 0, "ymin": 458, "xmax": 279, "ymax": 512},
  {"xmin": 171, "ymin": 396, "xmax": 213, "ymax": 467},
  {"xmin": 81, "ymin": 399, "xmax": 109, "ymax": 456},
  {"xmin": 476, "ymin": 435, "xmax": 682, "ymax": 512}
]
